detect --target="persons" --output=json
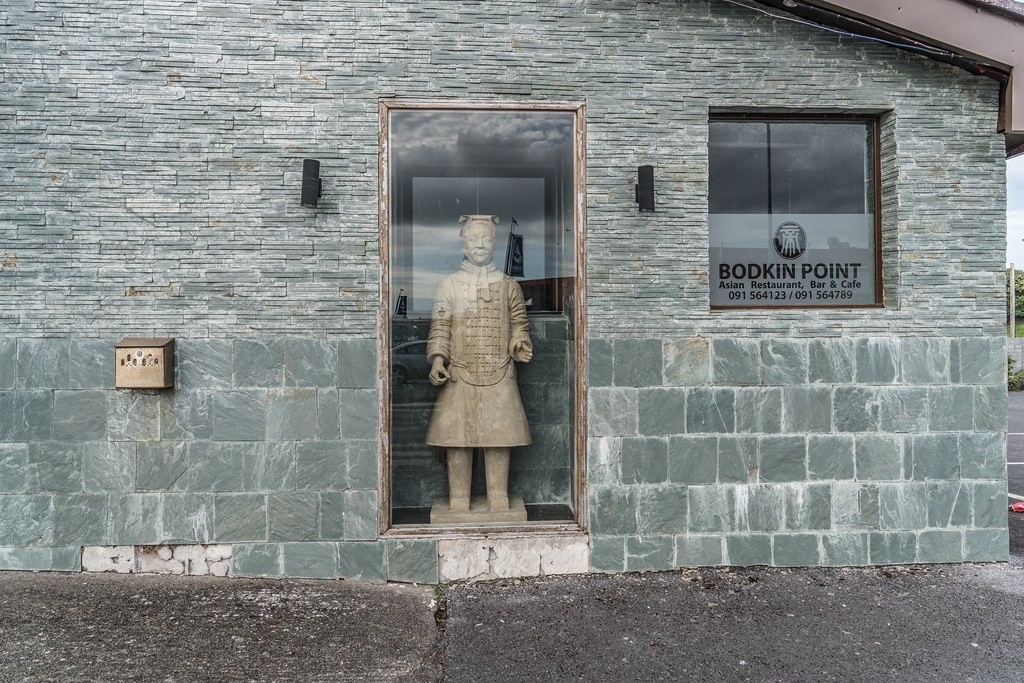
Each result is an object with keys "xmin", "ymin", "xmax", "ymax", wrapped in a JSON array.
[{"xmin": 423, "ymin": 213, "xmax": 534, "ymax": 517}]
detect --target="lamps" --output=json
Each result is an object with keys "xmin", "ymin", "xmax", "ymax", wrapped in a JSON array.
[
  {"xmin": 635, "ymin": 165, "xmax": 654, "ymax": 213},
  {"xmin": 300, "ymin": 159, "xmax": 323, "ymax": 208}
]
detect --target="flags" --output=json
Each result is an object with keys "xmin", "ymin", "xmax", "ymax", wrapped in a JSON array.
[
  {"xmin": 503, "ymin": 233, "xmax": 523, "ymax": 277},
  {"xmin": 394, "ymin": 295, "xmax": 406, "ymax": 316}
]
